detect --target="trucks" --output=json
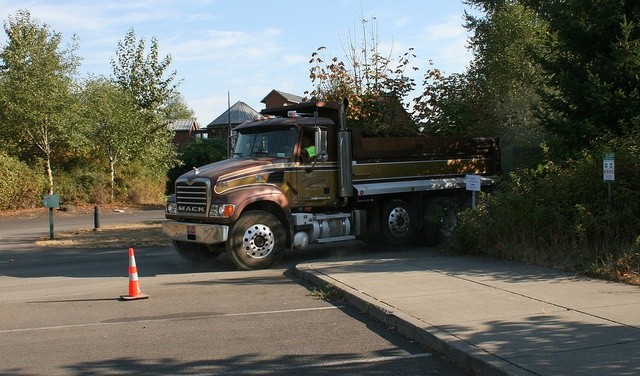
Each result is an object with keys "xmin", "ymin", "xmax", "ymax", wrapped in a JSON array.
[{"xmin": 162, "ymin": 97, "xmax": 500, "ymax": 271}]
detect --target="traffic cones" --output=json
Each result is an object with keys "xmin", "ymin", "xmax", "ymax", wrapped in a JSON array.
[{"xmin": 120, "ymin": 248, "xmax": 148, "ymax": 300}]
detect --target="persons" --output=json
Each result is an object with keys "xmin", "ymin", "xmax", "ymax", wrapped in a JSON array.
[{"xmin": 289, "ymin": 137, "xmax": 311, "ymax": 164}]
